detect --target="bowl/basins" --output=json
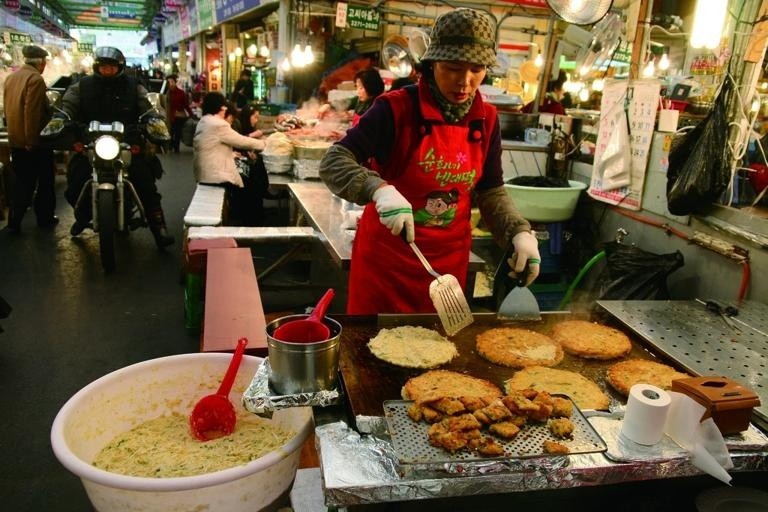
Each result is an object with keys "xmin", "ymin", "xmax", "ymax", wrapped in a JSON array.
[
  {"xmin": 658, "ymin": 100, "xmax": 688, "ymax": 113},
  {"xmin": 51, "ymin": 353, "xmax": 315, "ymax": 512},
  {"xmin": 497, "ymin": 111, "xmax": 539, "ymax": 139},
  {"xmin": 261, "ymin": 152, "xmax": 293, "ymax": 175},
  {"xmin": 471, "ymin": 177, "xmax": 587, "ymax": 231},
  {"xmin": 519, "ymin": 60, "xmax": 541, "ymax": 85},
  {"xmin": 293, "ymin": 141, "xmax": 332, "ymax": 159}
]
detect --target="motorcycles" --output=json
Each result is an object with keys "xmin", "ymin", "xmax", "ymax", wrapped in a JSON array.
[{"xmin": 38, "ymin": 80, "xmax": 180, "ymax": 269}]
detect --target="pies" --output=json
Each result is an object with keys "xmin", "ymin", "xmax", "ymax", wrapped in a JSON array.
[
  {"xmin": 476, "ymin": 327, "xmax": 564, "ymax": 369},
  {"xmin": 504, "ymin": 365, "xmax": 609, "ymax": 410},
  {"xmin": 604, "ymin": 358, "xmax": 691, "ymax": 397},
  {"xmin": 401, "ymin": 370, "xmax": 503, "ymax": 402},
  {"xmin": 551, "ymin": 321, "xmax": 633, "ymax": 360},
  {"xmin": 367, "ymin": 319, "xmax": 460, "ymax": 369}
]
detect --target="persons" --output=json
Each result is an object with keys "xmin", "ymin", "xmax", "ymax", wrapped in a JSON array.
[
  {"xmin": 166, "ymin": 74, "xmax": 193, "ymax": 154},
  {"xmin": 4, "ymin": 45, "xmax": 60, "ymax": 235},
  {"xmin": 318, "ymin": 7, "xmax": 542, "ymax": 316},
  {"xmin": 42, "ymin": 46, "xmax": 177, "ymax": 250},
  {"xmin": 328, "ymin": 65, "xmax": 383, "ymax": 128},
  {"xmin": 191, "ymin": 69, "xmax": 267, "ymax": 224}
]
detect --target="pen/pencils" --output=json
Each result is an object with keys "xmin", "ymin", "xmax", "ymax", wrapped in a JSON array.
[{"xmin": 659, "ymin": 96, "xmax": 675, "ymax": 111}]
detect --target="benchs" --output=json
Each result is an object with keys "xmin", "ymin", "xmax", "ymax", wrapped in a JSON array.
[
  {"xmin": 200, "ymin": 246, "xmax": 273, "ymax": 355},
  {"xmin": 184, "ymin": 224, "xmax": 315, "ymax": 301},
  {"xmin": 180, "ymin": 175, "xmax": 228, "ymax": 226}
]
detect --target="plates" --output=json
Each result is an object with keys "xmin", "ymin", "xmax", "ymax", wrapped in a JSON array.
[{"xmin": 345, "ymin": 210, "xmax": 364, "ymax": 237}]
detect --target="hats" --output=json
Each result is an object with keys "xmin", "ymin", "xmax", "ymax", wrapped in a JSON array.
[
  {"xmin": 419, "ymin": 9, "xmax": 500, "ymax": 68},
  {"xmin": 22, "ymin": 46, "xmax": 48, "ymax": 59}
]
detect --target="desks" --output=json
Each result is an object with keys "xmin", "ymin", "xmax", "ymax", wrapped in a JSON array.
[
  {"xmin": 285, "ymin": 183, "xmax": 485, "ymax": 305},
  {"xmin": 254, "ymin": 164, "xmax": 328, "ymax": 293}
]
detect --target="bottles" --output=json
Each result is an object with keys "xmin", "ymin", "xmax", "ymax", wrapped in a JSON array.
[{"xmin": 333, "ymin": 195, "xmax": 355, "ymax": 215}]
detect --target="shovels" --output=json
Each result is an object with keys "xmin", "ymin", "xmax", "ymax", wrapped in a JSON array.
[
  {"xmin": 400, "ymin": 222, "xmax": 474, "ymax": 337},
  {"xmin": 497, "ymin": 259, "xmax": 543, "ymax": 320}
]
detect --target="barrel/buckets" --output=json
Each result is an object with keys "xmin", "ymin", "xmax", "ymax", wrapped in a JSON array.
[
  {"xmin": 260, "ymin": 149, "xmax": 293, "ymax": 175},
  {"xmin": 264, "ymin": 313, "xmax": 342, "ymax": 396}
]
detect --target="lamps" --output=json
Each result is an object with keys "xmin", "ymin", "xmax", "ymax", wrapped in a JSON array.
[{"xmin": 279, "ymin": 1, "xmax": 315, "ymax": 73}]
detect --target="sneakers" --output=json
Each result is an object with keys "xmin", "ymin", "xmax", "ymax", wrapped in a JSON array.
[
  {"xmin": 2, "ymin": 226, "xmax": 20, "ymax": 233},
  {"xmin": 63, "ymin": 190, "xmax": 94, "ymax": 236},
  {"xmin": 38, "ymin": 216, "xmax": 60, "ymax": 230}
]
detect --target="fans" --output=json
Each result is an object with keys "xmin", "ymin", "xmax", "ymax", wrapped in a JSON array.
[
  {"xmin": 380, "ymin": 41, "xmax": 415, "ymax": 79},
  {"xmin": 407, "ymin": 26, "xmax": 430, "ymax": 63},
  {"xmin": 549, "ymin": 13, "xmax": 623, "ymax": 84},
  {"xmin": 543, "ymin": 0, "xmax": 630, "ymax": 28}
]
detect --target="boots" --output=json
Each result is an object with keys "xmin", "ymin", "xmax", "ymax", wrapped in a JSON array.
[{"xmin": 141, "ymin": 193, "xmax": 174, "ymax": 247}]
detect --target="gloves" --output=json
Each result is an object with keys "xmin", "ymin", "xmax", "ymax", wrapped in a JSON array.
[
  {"xmin": 40, "ymin": 112, "xmax": 73, "ymax": 151},
  {"xmin": 142, "ymin": 112, "xmax": 171, "ymax": 145},
  {"xmin": 372, "ymin": 184, "xmax": 415, "ymax": 244},
  {"xmin": 506, "ymin": 231, "xmax": 541, "ymax": 288}
]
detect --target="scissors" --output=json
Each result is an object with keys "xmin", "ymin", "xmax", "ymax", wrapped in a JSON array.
[{"xmin": 706, "ymin": 301, "xmax": 743, "ymax": 333}]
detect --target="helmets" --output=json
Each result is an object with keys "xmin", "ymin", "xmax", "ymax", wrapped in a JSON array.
[{"xmin": 92, "ymin": 47, "xmax": 127, "ymax": 82}]
